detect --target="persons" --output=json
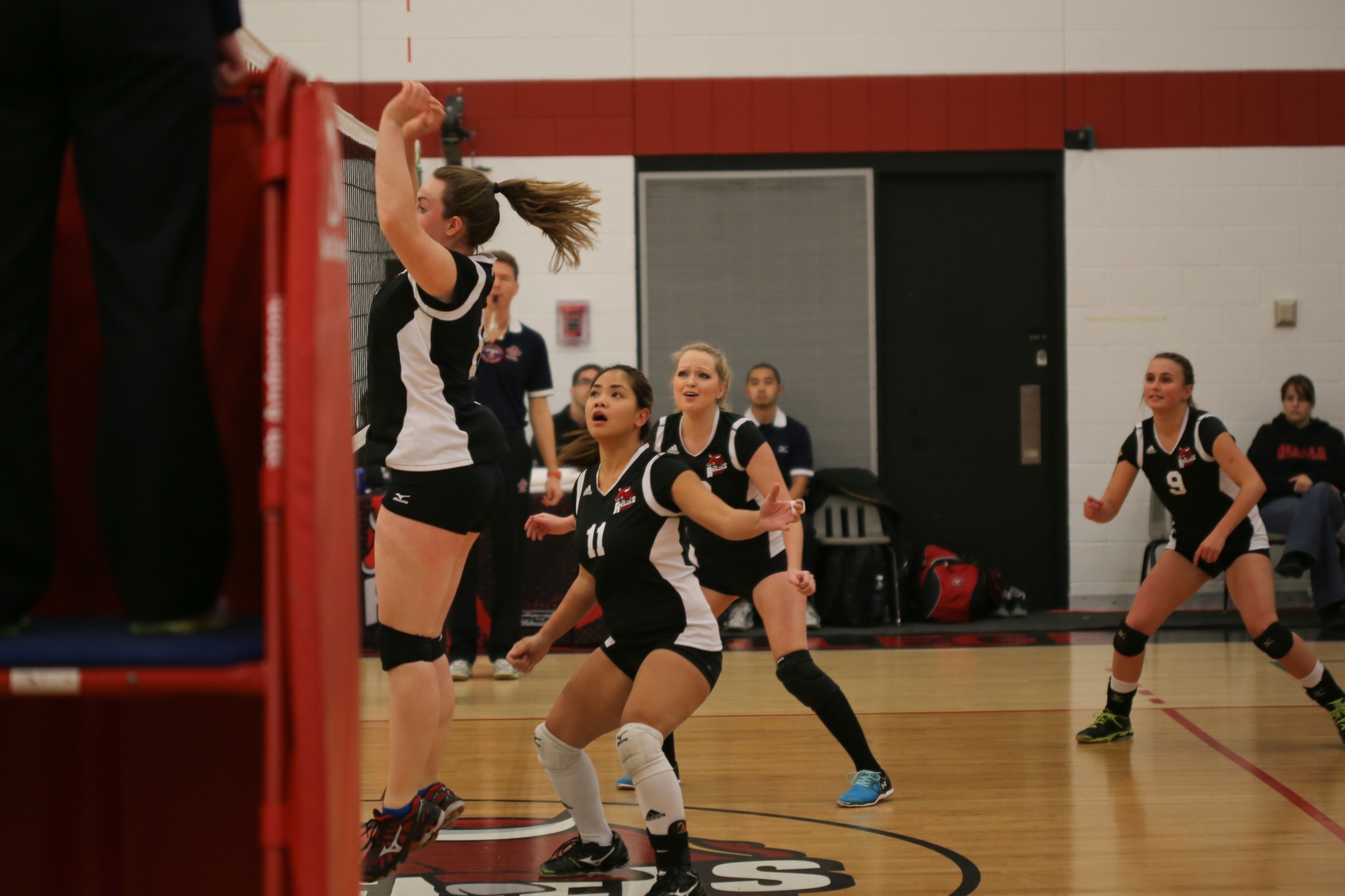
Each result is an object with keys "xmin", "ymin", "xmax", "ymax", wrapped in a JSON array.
[
  {"xmin": 1247, "ymin": 373, "xmax": 1345, "ymax": 637},
  {"xmin": 360, "ymin": 79, "xmax": 893, "ymax": 896},
  {"xmin": 1076, "ymin": 352, "xmax": 1344, "ymax": 744},
  {"xmin": 0, "ymin": 0, "xmax": 247, "ymax": 640}
]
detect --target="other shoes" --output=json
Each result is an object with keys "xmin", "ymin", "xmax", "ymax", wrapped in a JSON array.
[
  {"xmin": 1318, "ymin": 603, "xmax": 1345, "ymax": 640},
  {"xmin": 724, "ymin": 600, "xmax": 754, "ymax": 630},
  {"xmin": 491, "ymin": 657, "xmax": 519, "ymax": 679},
  {"xmin": 1274, "ymin": 552, "xmax": 1305, "ymax": 579},
  {"xmin": 449, "ymin": 659, "xmax": 473, "ymax": 679},
  {"xmin": 991, "ymin": 586, "xmax": 1028, "ymax": 617},
  {"xmin": 805, "ymin": 603, "xmax": 821, "ymax": 630}
]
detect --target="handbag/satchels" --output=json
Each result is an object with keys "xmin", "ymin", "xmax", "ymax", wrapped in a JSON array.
[{"xmin": 900, "ymin": 544, "xmax": 989, "ymax": 623}]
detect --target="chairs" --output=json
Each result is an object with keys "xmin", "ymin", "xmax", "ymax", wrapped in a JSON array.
[
  {"xmin": 805, "ymin": 468, "xmax": 907, "ymax": 626},
  {"xmin": 1138, "ymin": 488, "xmax": 1232, "ymax": 643}
]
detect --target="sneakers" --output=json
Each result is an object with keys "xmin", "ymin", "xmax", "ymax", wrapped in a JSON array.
[
  {"xmin": 1076, "ymin": 708, "xmax": 1133, "ymax": 743},
  {"xmin": 537, "ymin": 829, "xmax": 630, "ymax": 877},
  {"xmin": 615, "ymin": 759, "xmax": 681, "ymax": 789},
  {"xmin": 836, "ymin": 769, "xmax": 894, "ymax": 807},
  {"xmin": 359, "ymin": 781, "xmax": 465, "ymax": 884},
  {"xmin": 645, "ymin": 863, "xmax": 706, "ymax": 896},
  {"xmin": 1326, "ymin": 693, "xmax": 1345, "ymax": 741}
]
